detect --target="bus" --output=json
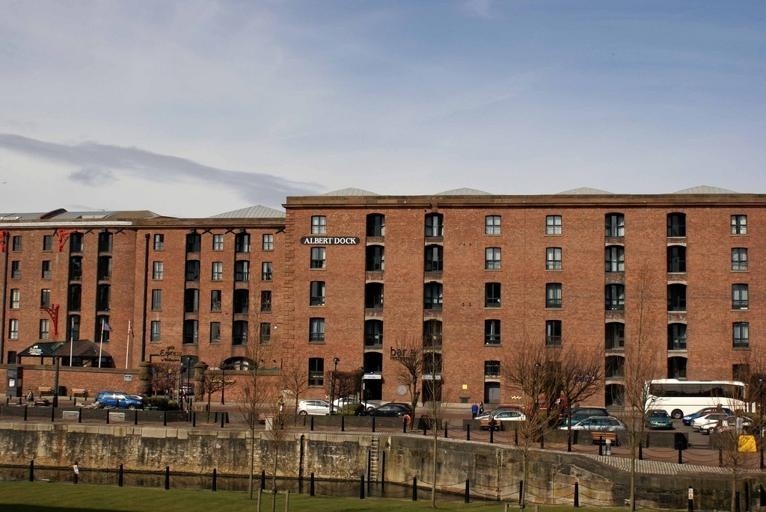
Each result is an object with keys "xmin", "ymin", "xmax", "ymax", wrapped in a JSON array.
[{"xmin": 644, "ymin": 379, "xmax": 761, "ymax": 419}]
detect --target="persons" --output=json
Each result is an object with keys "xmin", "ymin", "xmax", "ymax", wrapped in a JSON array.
[
  {"xmin": 470, "ymin": 400, "xmax": 478, "ymax": 419},
  {"xmin": 72, "ymin": 460, "xmax": 79, "ymax": 484},
  {"xmin": 274, "ymin": 394, "xmax": 284, "ymax": 411},
  {"xmin": 479, "ymin": 400, "xmax": 484, "ymax": 414}
]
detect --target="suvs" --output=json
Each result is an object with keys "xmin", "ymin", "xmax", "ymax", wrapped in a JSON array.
[
  {"xmin": 558, "ymin": 416, "xmax": 627, "ymax": 432},
  {"xmin": 557, "ymin": 406, "xmax": 611, "ymax": 426},
  {"xmin": 94, "ymin": 388, "xmax": 146, "ymax": 409}
]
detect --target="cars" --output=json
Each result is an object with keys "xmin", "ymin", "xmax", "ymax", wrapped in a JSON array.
[
  {"xmin": 644, "ymin": 409, "xmax": 673, "ymax": 430},
  {"xmin": 296, "ymin": 399, "xmax": 338, "ymax": 416},
  {"xmin": 368, "ymin": 402, "xmax": 412, "ymax": 417},
  {"xmin": 329, "ymin": 397, "xmax": 377, "ymax": 413},
  {"xmin": 682, "ymin": 407, "xmax": 754, "ymax": 435},
  {"xmin": 473, "ymin": 404, "xmax": 526, "ymax": 422}
]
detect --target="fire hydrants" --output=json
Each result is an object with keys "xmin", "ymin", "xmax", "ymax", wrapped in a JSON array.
[{"xmin": 603, "ymin": 438, "xmax": 612, "ymax": 456}]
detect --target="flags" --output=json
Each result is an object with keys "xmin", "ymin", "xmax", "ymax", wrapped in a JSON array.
[
  {"xmin": 128, "ymin": 321, "xmax": 134, "ymax": 337},
  {"xmin": 103, "ymin": 320, "xmax": 112, "ymax": 331}
]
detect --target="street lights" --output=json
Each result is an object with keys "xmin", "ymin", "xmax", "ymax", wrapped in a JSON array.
[
  {"xmin": 533, "ymin": 360, "xmax": 541, "ymax": 414},
  {"xmin": 184, "ymin": 354, "xmax": 193, "ymax": 415},
  {"xmin": 328, "ymin": 356, "xmax": 340, "ymax": 415}
]
